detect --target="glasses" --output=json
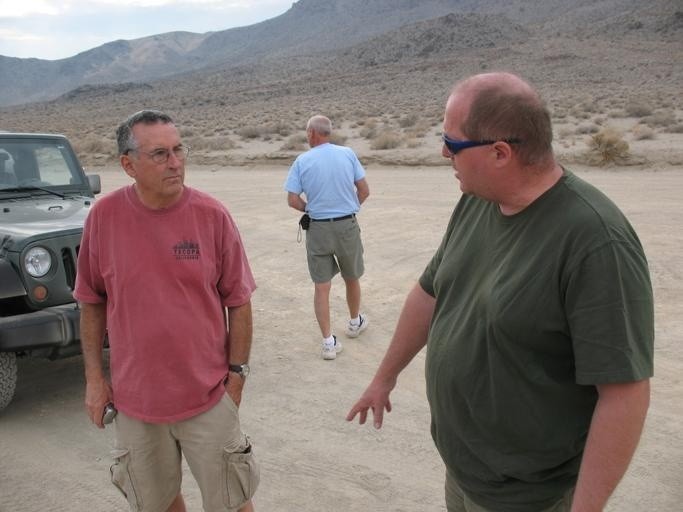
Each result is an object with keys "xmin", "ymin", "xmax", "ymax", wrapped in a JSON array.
[
  {"xmin": 123, "ymin": 143, "xmax": 190, "ymax": 164},
  {"xmin": 441, "ymin": 133, "xmax": 521, "ymax": 156}
]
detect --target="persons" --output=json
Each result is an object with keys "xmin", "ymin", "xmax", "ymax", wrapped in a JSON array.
[
  {"xmin": 343, "ymin": 71, "xmax": 658, "ymax": 511},
  {"xmin": 282, "ymin": 114, "xmax": 371, "ymax": 361},
  {"xmin": 69, "ymin": 109, "xmax": 262, "ymax": 511}
]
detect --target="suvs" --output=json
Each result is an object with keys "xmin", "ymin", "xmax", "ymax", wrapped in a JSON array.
[{"xmin": 0, "ymin": 131, "xmax": 109, "ymax": 409}]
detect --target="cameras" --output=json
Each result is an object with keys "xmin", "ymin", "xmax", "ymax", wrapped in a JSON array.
[{"xmin": 102, "ymin": 403, "xmax": 116, "ymax": 425}]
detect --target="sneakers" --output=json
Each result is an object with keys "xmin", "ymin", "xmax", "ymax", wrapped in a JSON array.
[
  {"xmin": 347, "ymin": 314, "xmax": 369, "ymax": 338},
  {"xmin": 322, "ymin": 333, "xmax": 343, "ymax": 360}
]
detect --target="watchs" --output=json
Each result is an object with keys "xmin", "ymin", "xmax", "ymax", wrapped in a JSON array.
[{"xmin": 225, "ymin": 362, "xmax": 250, "ymax": 379}]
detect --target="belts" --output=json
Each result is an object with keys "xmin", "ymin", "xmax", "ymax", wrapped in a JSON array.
[{"xmin": 310, "ymin": 215, "xmax": 356, "ymax": 222}]
